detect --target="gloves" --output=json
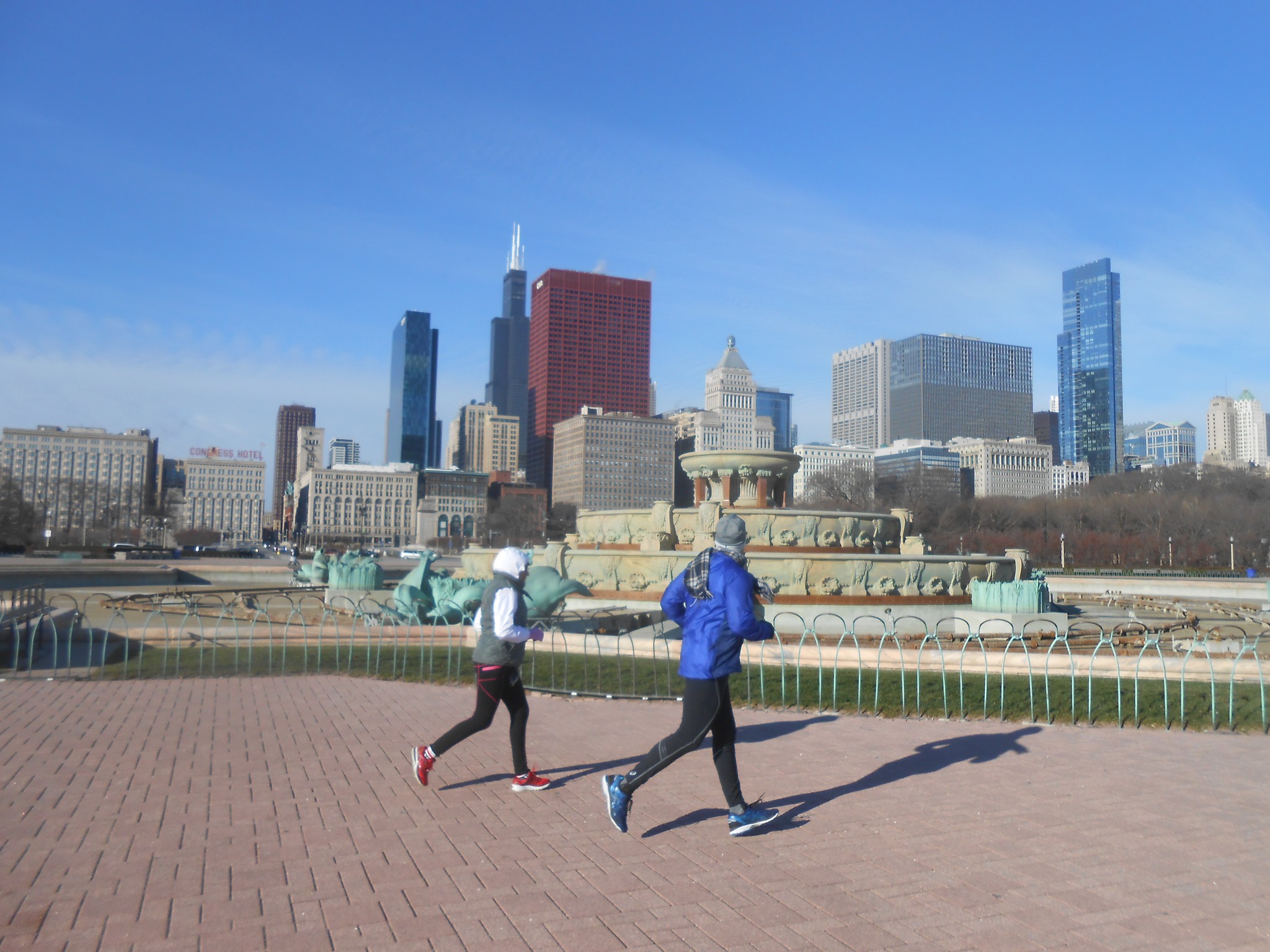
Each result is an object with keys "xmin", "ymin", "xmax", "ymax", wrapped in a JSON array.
[
  {"xmin": 762, "ymin": 620, "xmax": 774, "ymax": 639},
  {"xmin": 528, "ymin": 627, "xmax": 544, "ymax": 641}
]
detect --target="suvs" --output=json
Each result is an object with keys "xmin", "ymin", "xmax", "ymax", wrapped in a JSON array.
[{"xmin": 400, "ymin": 550, "xmax": 441, "ymax": 560}]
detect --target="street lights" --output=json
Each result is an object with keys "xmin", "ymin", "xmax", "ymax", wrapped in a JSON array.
[
  {"xmin": 1168, "ymin": 536, "xmax": 1173, "ymax": 567},
  {"xmin": 1228, "ymin": 536, "xmax": 1235, "ymax": 570},
  {"xmin": 46, "ymin": 511, "xmax": 52, "ymax": 547},
  {"xmin": 960, "ymin": 536, "xmax": 964, "ymax": 553},
  {"xmin": 1060, "ymin": 533, "xmax": 1066, "ymax": 568},
  {"xmin": 162, "ymin": 518, "xmax": 168, "ymax": 548}
]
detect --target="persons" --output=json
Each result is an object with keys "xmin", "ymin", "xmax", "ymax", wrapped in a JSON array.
[
  {"xmin": 411, "ymin": 547, "xmax": 552, "ymax": 792},
  {"xmin": 602, "ymin": 513, "xmax": 779, "ymax": 836},
  {"xmin": 287, "ymin": 546, "xmax": 300, "ymax": 565}
]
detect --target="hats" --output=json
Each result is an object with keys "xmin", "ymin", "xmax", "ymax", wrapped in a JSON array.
[
  {"xmin": 492, "ymin": 547, "xmax": 530, "ymax": 580},
  {"xmin": 715, "ymin": 515, "xmax": 746, "ymax": 552}
]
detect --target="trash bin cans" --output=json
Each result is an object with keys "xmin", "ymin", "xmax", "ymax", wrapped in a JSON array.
[
  {"xmin": 173, "ymin": 549, "xmax": 181, "ymax": 559},
  {"xmin": 1246, "ymin": 568, "xmax": 1256, "ymax": 578}
]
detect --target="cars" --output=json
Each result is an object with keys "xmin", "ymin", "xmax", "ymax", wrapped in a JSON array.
[
  {"xmin": 277, "ymin": 546, "xmax": 284, "ymax": 552},
  {"xmin": 358, "ymin": 549, "xmax": 380, "ymax": 558},
  {"xmin": 113, "ymin": 543, "xmax": 135, "ymax": 548}
]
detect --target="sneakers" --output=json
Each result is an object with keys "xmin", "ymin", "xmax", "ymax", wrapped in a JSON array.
[
  {"xmin": 411, "ymin": 746, "xmax": 436, "ymax": 786},
  {"xmin": 727, "ymin": 792, "xmax": 779, "ymax": 836},
  {"xmin": 511, "ymin": 764, "xmax": 550, "ymax": 791},
  {"xmin": 602, "ymin": 775, "xmax": 633, "ymax": 833}
]
